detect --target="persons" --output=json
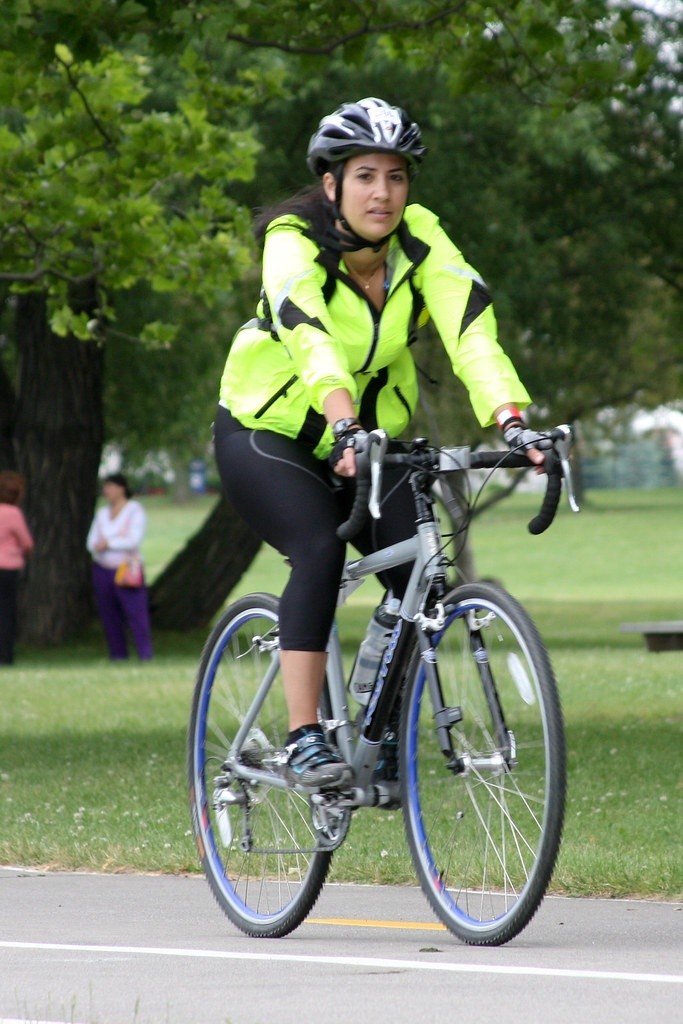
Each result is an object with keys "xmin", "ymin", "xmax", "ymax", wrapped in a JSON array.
[
  {"xmin": 214, "ymin": 98, "xmax": 571, "ymax": 795},
  {"xmin": 86, "ymin": 475, "xmax": 153, "ymax": 663},
  {"xmin": 0, "ymin": 471, "xmax": 34, "ymax": 666}
]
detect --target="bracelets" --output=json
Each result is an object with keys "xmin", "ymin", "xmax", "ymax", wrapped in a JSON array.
[{"xmin": 495, "ymin": 408, "xmax": 525, "ymax": 431}]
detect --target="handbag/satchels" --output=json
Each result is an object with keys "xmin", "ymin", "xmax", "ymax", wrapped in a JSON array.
[{"xmin": 114, "ymin": 550, "xmax": 143, "ymax": 590}]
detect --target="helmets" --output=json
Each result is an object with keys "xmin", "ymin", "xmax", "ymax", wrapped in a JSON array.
[{"xmin": 306, "ymin": 96, "xmax": 432, "ymax": 179}]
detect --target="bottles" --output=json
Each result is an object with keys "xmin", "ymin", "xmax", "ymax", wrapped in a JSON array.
[{"xmin": 350, "ymin": 599, "xmax": 403, "ymax": 705}]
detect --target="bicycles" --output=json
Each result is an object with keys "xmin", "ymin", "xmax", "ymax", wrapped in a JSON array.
[{"xmin": 185, "ymin": 423, "xmax": 583, "ymax": 945}]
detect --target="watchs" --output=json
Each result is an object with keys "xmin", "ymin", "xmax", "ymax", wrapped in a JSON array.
[{"xmin": 332, "ymin": 417, "xmax": 360, "ymax": 439}]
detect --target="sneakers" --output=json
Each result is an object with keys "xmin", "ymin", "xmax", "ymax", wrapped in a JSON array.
[
  {"xmin": 283, "ymin": 727, "xmax": 356, "ymax": 795},
  {"xmin": 354, "ymin": 707, "xmax": 405, "ymax": 811}
]
